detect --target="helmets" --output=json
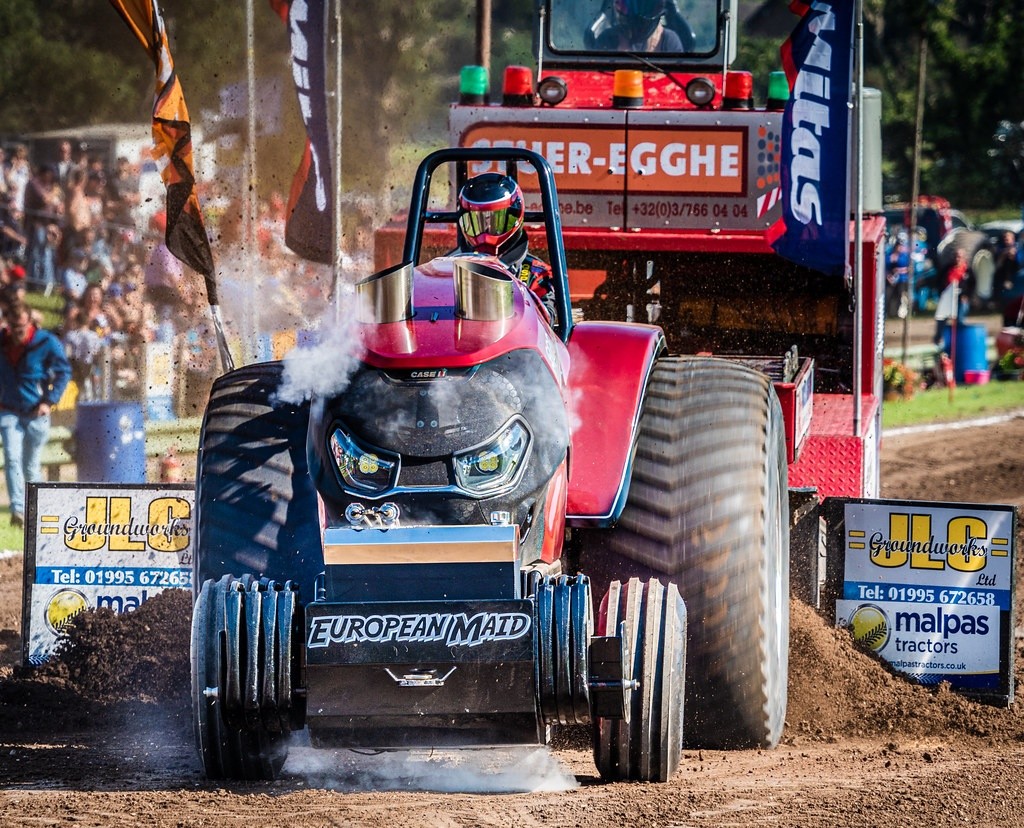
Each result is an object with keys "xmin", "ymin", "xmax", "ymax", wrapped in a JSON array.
[
  {"xmin": 614, "ymin": 0, "xmax": 665, "ymax": 43},
  {"xmin": 457, "ymin": 172, "xmax": 526, "ymax": 255}
]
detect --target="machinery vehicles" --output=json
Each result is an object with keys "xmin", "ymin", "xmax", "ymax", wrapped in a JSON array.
[
  {"xmin": 371, "ymin": 0, "xmax": 887, "ymax": 607},
  {"xmin": 190, "ymin": 146, "xmax": 789, "ymax": 785}
]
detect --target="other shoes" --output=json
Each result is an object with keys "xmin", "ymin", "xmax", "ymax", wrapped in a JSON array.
[{"xmin": 10, "ymin": 512, "xmax": 24, "ymax": 530}]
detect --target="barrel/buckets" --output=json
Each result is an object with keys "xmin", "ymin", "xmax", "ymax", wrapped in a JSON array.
[
  {"xmin": 944, "ymin": 325, "xmax": 986, "ymax": 386},
  {"xmin": 66, "ymin": 401, "xmax": 148, "ymax": 484}
]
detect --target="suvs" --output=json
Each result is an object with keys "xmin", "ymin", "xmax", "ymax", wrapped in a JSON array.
[{"xmin": 883, "ymin": 208, "xmax": 971, "ymax": 259}]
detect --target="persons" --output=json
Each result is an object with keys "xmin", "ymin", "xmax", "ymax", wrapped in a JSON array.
[
  {"xmin": 442, "ymin": 173, "xmax": 556, "ymax": 331},
  {"xmin": 0, "ymin": 142, "xmax": 378, "ymax": 529},
  {"xmin": 884, "ymin": 211, "xmax": 1024, "ymax": 344},
  {"xmin": 593, "ymin": 0, "xmax": 684, "ymax": 53}
]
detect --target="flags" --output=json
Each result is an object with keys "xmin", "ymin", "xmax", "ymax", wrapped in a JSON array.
[
  {"xmin": 769, "ymin": 0, "xmax": 854, "ymax": 278},
  {"xmin": 269, "ymin": 0, "xmax": 335, "ymax": 265},
  {"xmin": 110, "ymin": 0, "xmax": 218, "ymax": 305}
]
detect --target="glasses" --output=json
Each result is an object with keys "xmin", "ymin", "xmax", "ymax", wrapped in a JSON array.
[{"xmin": 12, "ymin": 322, "xmax": 28, "ymax": 328}]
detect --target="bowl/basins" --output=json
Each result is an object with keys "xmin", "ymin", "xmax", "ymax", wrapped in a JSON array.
[{"xmin": 964, "ymin": 371, "xmax": 990, "ymax": 384}]
